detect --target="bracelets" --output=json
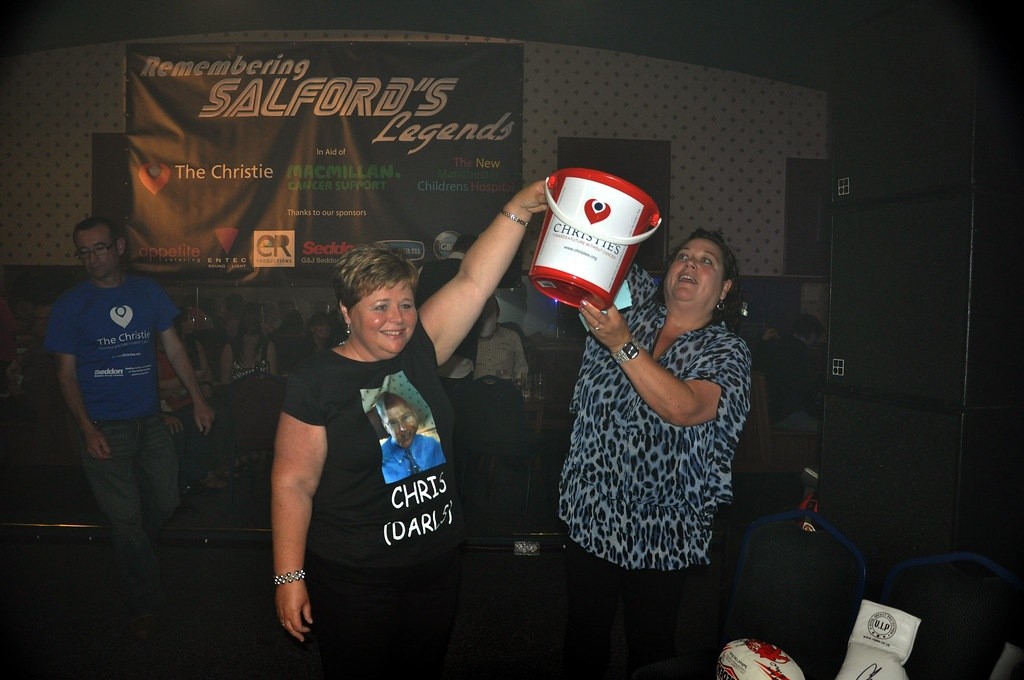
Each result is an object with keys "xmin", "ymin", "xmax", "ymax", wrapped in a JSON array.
[
  {"xmin": 499, "ymin": 208, "xmax": 528, "ymax": 228},
  {"xmin": 273, "ymin": 569, "xmax": 305, "ymax": 585}
]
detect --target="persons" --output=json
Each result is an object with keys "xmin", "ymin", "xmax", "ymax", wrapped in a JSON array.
[
  {"xmin": 0, "ymin": 215, "xmax": 828, "ymax": 680},
  {"xmin": 271, "ymin": 178, "xmax": 549, "ymax": 680}
]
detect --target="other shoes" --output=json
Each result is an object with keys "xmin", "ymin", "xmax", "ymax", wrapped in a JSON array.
[
  {"xmin": 127, "ymin": 596, "xmax": 166, "ymax": 638},
  {"xmin": 202, "ymin": 462, "xmax": 247, "ymax": 489}
]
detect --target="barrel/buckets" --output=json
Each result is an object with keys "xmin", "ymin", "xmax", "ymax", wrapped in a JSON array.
[{"xmin": 528, "ymin": 168, "xmax": 664, "ymax": 311}]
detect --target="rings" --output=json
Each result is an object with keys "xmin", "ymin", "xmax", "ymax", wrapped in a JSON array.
[{"xmin": 594, "ymin": 322, "xmax": 601, "ymax": 330}]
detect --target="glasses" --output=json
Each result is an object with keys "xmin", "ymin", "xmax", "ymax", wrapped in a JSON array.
[{"xmin": 76, "ymin": 240, "xmax": 116, "ymax": 259}]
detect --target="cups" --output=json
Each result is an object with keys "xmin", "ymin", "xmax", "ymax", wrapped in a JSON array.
[
  {"xmin": 520, "ymin": 372, "xmax": 533, "ymax": 401},
  {"xmin": 535, "ymin": 373, "xmax": 546, "ymax": 400},
  {"xmin": 494, "ymin": 369, "xmax": 509, "ymax": 379}
]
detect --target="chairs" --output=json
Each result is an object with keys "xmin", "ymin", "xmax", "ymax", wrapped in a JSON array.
[
  {"xmin": 0, "ymin": 304, "xmax": 821, "ymax": 525},
  {"xmin": 872, "ymin": 552, "xmax": 1024, "ymax": 680},
  {"xmin": 634, "ymin": 508, "xmax": 867, "ymax": 680}
]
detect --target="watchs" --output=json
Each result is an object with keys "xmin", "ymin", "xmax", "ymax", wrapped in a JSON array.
[{"xmin": 611, "ymin": 335, "xmax": 642, "ymax": 365}]
